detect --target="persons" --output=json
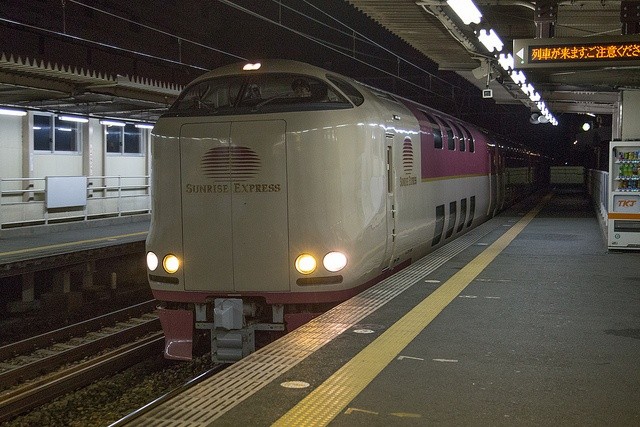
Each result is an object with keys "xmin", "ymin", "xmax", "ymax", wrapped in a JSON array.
[{"xmin": 291, "ymin": 79, "xmax": 311, "ymax": 97}]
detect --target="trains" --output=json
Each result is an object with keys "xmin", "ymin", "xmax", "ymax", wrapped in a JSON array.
[{"xmin": 143, "ymin": 58, "xmax": 530, "ymax": 369}]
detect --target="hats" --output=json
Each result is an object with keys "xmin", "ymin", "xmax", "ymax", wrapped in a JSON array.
[{"xmin": 291, "ymin": 79, "xmax": 311, "ymax": 91}]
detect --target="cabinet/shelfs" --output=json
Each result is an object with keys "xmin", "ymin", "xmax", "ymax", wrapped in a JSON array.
[{"xmin": 609, "ymin": 141, "xmax": 640, "ymax": 250}]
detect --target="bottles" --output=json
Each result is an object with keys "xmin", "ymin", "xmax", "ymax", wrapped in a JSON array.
[{"xmin": 618, "ymin": 150, "xmax": 640, "ymax": 190}]
